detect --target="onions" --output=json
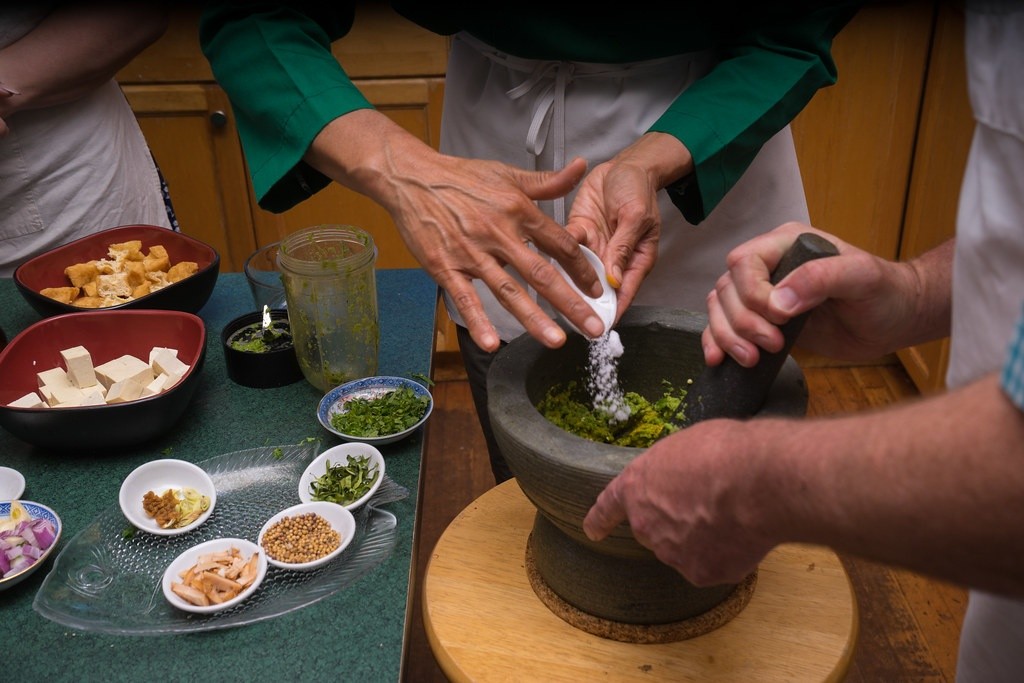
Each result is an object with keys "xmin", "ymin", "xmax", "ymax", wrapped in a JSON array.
[{"xmin": 0, "ymin": 517, "xmax": 56, "ymax": 581}]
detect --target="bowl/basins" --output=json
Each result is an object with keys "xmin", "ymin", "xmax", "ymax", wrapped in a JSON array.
[
  {"xmin": 550, "ymin": 243, "xmax": 620, "ymax": 342},
  {"xmin": 118, "ymin": 458, "xmax": 217, "ymax": 535},
  {"xmin": 13, "ymin": 222, "xmax": 221, "ymax": 319},
  {"xmin": 297, "ymin": 441, "xmax": 387, "ymax": 510},
  {"xmin": 257, "ymin": 501, "xmax": 357, "ymax": 572},
  {"xmin": 317, "ymin": 376, "xmax": 434, "ymax": 446},
  {"xmin": 161, "ymin": 538, "xmax": 267, "ymax": 612},
  {"xmin": 0, "ymin": 310, "xmax": 209, "ymax": 439},
  {"xmin": 221, "ymin": 308, "xmax": 306, "ymax": 389}
]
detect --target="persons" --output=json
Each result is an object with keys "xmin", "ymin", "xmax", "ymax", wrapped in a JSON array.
[
  {"xmin": 582, "ymin": 0, "xmax": 1024, "ymax": 683},
  {"xmin": 196, "ymin": 0, "xmax": 865, "ymax": 486},
  {"xmin": 0, "ymin": 0, "xmax": 181, "ymax": 278}
]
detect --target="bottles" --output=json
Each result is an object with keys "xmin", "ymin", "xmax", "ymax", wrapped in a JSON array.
[{"xmin": 276, "ymin": 224, "xmax": 380, "ymax": 391}]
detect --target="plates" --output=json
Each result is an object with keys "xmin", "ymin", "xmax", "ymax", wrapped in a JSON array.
[
  {"xmin": 0, "ymin": 500, "xmax": 62, "ymax": 589},
  {"xmin": 0, "ymin": 466, "xmax": 28, "ymax": 502},
  {"xmin": 34, "ymin": 445, "xmax": 408, "ymax": 636}
]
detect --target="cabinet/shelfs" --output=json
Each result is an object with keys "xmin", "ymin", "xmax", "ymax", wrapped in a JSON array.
[{"xmin": 122, "ymin": 78, "xmax": 460, "ymax": 352}]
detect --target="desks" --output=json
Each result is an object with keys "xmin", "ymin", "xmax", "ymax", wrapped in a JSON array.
[
  {"xmin": 0, "ymin": 266, "xmax": 441, "ymax": 683},
  {"xmin": 422, "ymin": 477, "xmax": 859, "ymax": 683}
]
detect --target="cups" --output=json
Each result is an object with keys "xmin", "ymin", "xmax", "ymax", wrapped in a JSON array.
[{"xmin": 242, "ymin": 239, "xmax": 290, "ymax": 311}]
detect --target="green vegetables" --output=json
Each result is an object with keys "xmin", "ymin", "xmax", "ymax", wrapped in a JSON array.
[
  {"xmin": 329, "ymin": 385, "xmax": 430, "ymax": 438},
  {"xmin": 308, "ymin": 454, "xmax": 379, "ymax": 506},
  {"xmin": 409, "ymin": 372, "xmax": 435, "ymax": 387}
]
